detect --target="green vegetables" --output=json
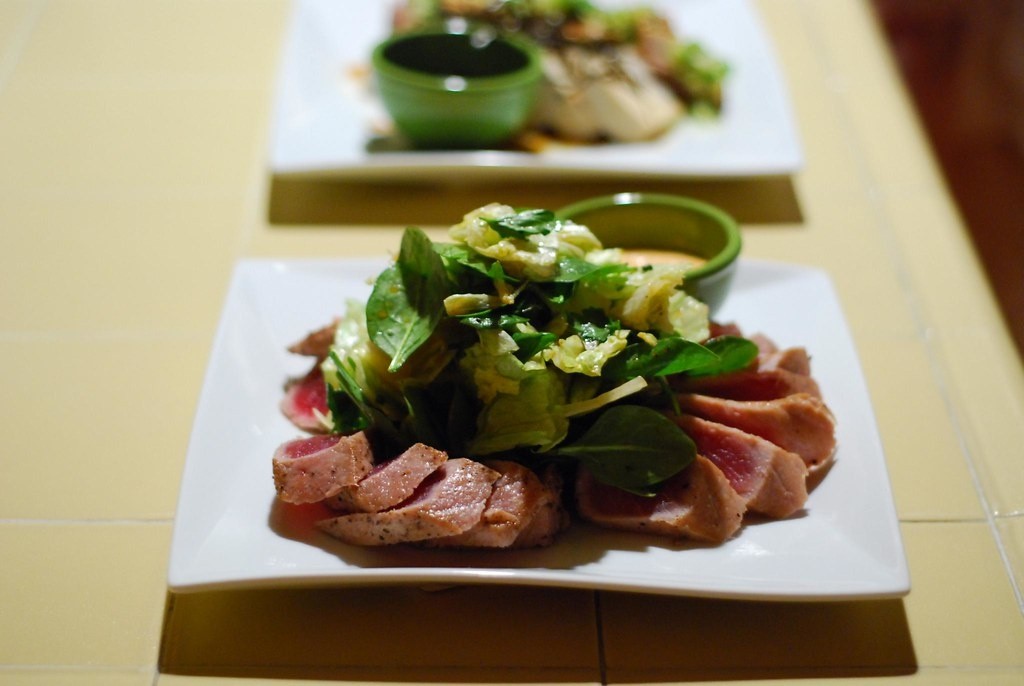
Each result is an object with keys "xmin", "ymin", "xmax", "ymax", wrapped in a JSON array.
[{"xmin": 327, "ymin": 207, "xmax": 757, "ymax": 484}]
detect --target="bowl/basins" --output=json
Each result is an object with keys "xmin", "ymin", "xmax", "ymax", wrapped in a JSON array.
[
  {"xmin": 557, "ymin": 194, "xmax": 742, "ymax": 323},
  {"xmin": 372, "ymin": 30, "xmax": 540, "ymax": 146}
]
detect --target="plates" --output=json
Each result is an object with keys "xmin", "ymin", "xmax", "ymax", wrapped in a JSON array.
[
  {"xmin": 265, "ymin": 0, "xmax": 804, "ymax": 178},
  {"xmin": 166, "ymin": 255, "xmax": 910, "ymax": 598}
]
